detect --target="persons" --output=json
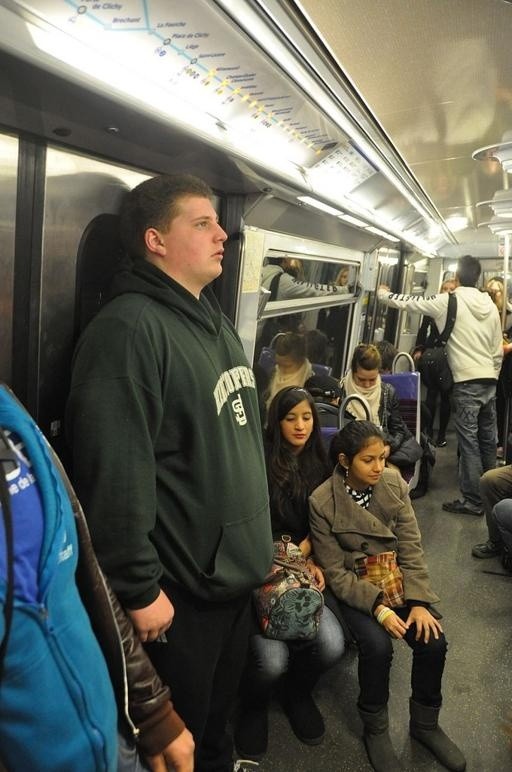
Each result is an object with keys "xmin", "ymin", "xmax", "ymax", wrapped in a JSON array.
[
  {"xmin": 309, "ymin": 420, "xmax": 466, "ymax": 772},
  {"xmin": 377, "ymin": 254, "xmax": 511, "ymax": 571},
  {"xmin": 0, "ymin": 381, "xmax": 196, "ymax": 772},
  {"xmin": 258, "ymin": 257, "xmax": 350, "ymax": 406},
  {"xmin": 66, "ymin": 174, "xmax": 273, "ymax": 771},
  {"xmin": 338, "ymin": 343, "xmax": 406, "ymax": 465},
  {"xmin": 233, "ymin": 385, "xmax": 347, "ymax": 761}
]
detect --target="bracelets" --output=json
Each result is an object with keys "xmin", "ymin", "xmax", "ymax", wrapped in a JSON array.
[{"xmin": 377, "ymin": 606, "xmax": 391, "ymax": 623}]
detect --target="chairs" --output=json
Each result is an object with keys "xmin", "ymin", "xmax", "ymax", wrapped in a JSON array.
[
  {"xmin": 257, "ymin": 346, "xmax": 332, "ymax": 380},
  {"xmin": 312, "ymin": 351, "xmax": 421, "ymax": 496}
]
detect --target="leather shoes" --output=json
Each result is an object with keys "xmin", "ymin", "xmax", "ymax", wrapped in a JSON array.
[
  {"xmin": 436, "ymin": 431, "xmax": 447, "ymax": 447},
  {"xmin": 442, "ymin": 500, "xmax": 484, "ymax": 516},
  {"xmin": 471, "ymin": 540, "xmax": 497, "ymax": 559}
]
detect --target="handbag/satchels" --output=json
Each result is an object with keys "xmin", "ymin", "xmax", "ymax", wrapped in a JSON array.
[
  {"xmin": 380, "ymin": 429, "xmax": 403, "ymax": 449},
  {"xmin": 252, "ymin": 540, "xmax": 324, "ymax": 640}
]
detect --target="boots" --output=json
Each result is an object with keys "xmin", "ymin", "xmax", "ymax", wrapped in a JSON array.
[
  {"xmin": 233, "ymin": 664, "xmax": 269, "ymax": 761},
  {"xmin": 356, "ymin": 702, "xmax": 405, "ymax": 772},
  {"xmin": 409, "ymin": 698, "xmax": 465, "ymax": 772},
  {"xmin": 274, "ymin": 655, "xmax": 325, "ymax": 746}
]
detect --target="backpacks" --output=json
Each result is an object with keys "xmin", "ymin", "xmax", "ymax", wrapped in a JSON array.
[{"xmin": 418, "ymin": 289, "xmax": 457, "ymax": 394}]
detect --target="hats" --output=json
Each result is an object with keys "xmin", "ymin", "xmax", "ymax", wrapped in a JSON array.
[{"xmin": 304, "ymin": 375, "xmax": 345, "ymax": 398}]
